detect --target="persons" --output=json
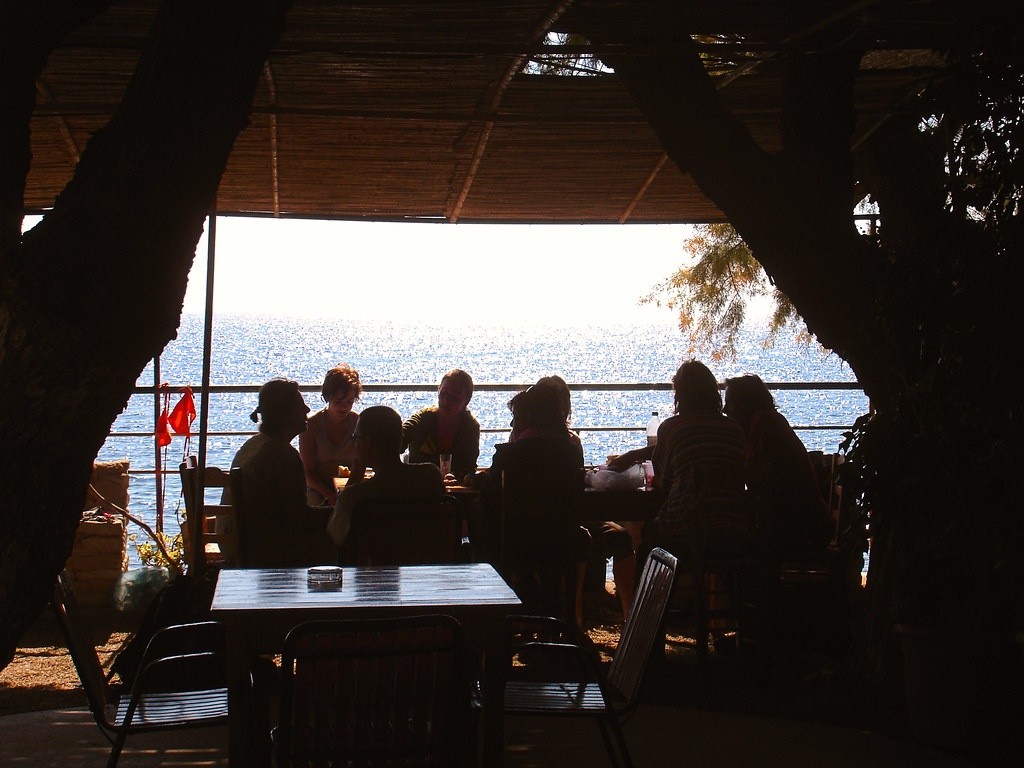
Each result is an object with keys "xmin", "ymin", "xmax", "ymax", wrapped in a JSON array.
[
  {"xmin": 300, "ymin": 369, "xmax": 361, "ymax": 508},
  {"xmin": 610, "ymin": 361, "xmax": 831, "ymax": 661},
  {"xmin": 328, "ymin": 406, "xmax": 445, "ymax": 566},
  {"xmin": 400, "ymin": 371, "xmax": 479, "ymax": 482},
  {"xmin": 470, "ymin": 376, "xmax": 593, "ymax": 664},
  {"xmin": 214, "ymin": 380, "xmax": 333, "ymax": 569}
]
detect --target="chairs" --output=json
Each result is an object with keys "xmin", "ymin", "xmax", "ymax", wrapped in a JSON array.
[
  {"xmin": 47, "ymin": 565, "xmax": 230, "ymax": 767},
  {"xmin": 180, "ymin": 463, "xmax": 243, "ymax": 573},
  {"xmin": 504, "ymin": 545, "xmax": 683, "ymax": 767},
  {"xmin": 495, "ymin": 443, "xmax": 583, "ymax": 572},
  {"xmin": 664, "ymin": 465, "xmax": 754, "ymax": 671},
  {"xmin": 267, "ymin": 615, "xmax": 481, "ymax": 767},
  {"xmin": 769, "ymin": 450, "xmax": 877, "ymax": 595},
  {"xmin": 346, "ymin": 494, "xmax": 459, "ymax": 565}
]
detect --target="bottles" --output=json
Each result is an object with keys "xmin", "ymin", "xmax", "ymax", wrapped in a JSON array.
[{"xmin": 646, "ymin": 412, "xmax": 661, "ymax": 448}]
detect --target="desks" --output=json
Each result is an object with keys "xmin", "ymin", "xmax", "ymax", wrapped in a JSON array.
[
  {"xmin": 209, "ymin": 562, "xmax": 523, "ymax": 768},
  {"xmin": 321, "ymin": 491, "xmax": 653, "ymax": 522}
]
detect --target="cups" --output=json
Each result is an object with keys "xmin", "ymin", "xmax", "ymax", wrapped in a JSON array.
[
  {"xmin": 642, "ymin": 463, "xmax": 655, "ymax": 483},
  {"xmin": 440, "ymin": 453, "xmax": 452, "ymax": 478}
]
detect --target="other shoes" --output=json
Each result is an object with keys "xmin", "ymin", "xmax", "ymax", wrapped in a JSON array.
[
  {"xmin": 581, "ymin": 591, "xmax": 625, "ymax": 623},
  {"xmin": 647, "ymin": 636, "xmax": 675, "ymax": 664},
  {"xmin": 714, "ymin": 621, "xmax": 775, "ymax": 655}
]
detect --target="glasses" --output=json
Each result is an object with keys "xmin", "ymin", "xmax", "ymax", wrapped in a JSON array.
[
  {"xmin": 351, "ymin": 433, "xmax": 380, "ymax": 450},
  {"xmin": 525, "ymin": 386, "xmax": 540, "ymax": 408}
]
